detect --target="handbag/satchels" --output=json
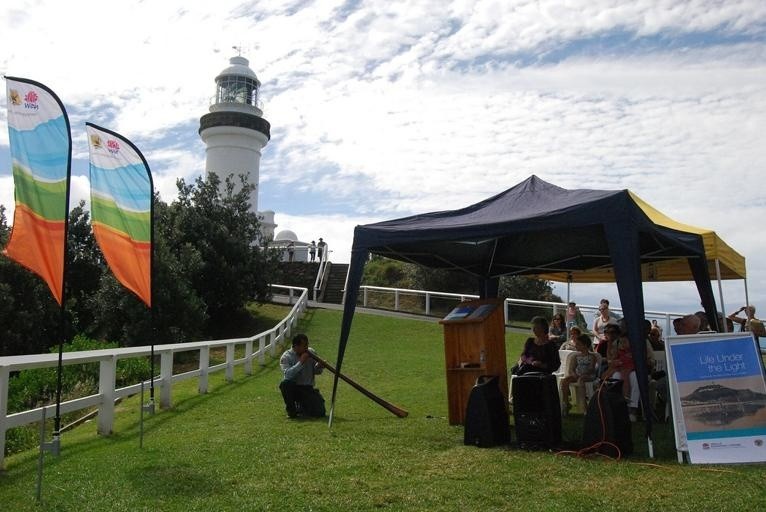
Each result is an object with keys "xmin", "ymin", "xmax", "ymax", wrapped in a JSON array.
[{"xmin": 512, "ymin": 357, "xmax": 538, "ymax": 376}]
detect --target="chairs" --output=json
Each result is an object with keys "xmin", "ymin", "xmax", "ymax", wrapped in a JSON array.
[{"xmin": 511, "ymin": 341, "xmax": 668, "ymax": 416}]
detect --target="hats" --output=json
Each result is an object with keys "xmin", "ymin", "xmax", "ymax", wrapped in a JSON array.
[{"xmin": 570, "ymin": 300, "xmax": 576, "ymax": 305}]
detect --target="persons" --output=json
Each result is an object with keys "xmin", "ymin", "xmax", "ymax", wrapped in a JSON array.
[
  {"xmin": 308, "ymin": 241, "xmax": 316, "ymax": 262},
  {"xmin": 318, "ymin": 238, "xmax": 326, "ymax": 262},
  {"xmin": 280, "ymin": 334, "xmax": 326, "ymax": 418},
  {"xmin": 509, "ymin": 299, "xmax": 765, "ymax": 423},
  {"xmin": 287, "ymin": 242, "xmax": 294, "ymax": 262}
]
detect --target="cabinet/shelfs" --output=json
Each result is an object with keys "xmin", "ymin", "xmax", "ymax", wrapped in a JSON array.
[{"xmin": 438, "ymin": 299, "xmax": 511, "ymax": 426}]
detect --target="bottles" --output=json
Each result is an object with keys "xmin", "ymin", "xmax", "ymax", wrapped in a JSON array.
[{"xmin": 480, "ymin": 350, "xmax": 488, "ymax": 371}]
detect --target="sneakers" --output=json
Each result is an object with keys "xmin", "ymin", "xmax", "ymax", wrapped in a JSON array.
[
  {"xmin": 563, "ymin": 403, "xmax": 572, "ymax": 416},
  {"xmin": 286, "ymin": 401, "xmax": 302, "ymax": 419},
  {"xmin": 629, "ymin": 414, "xmax": 637, "ymax": 422}
]
